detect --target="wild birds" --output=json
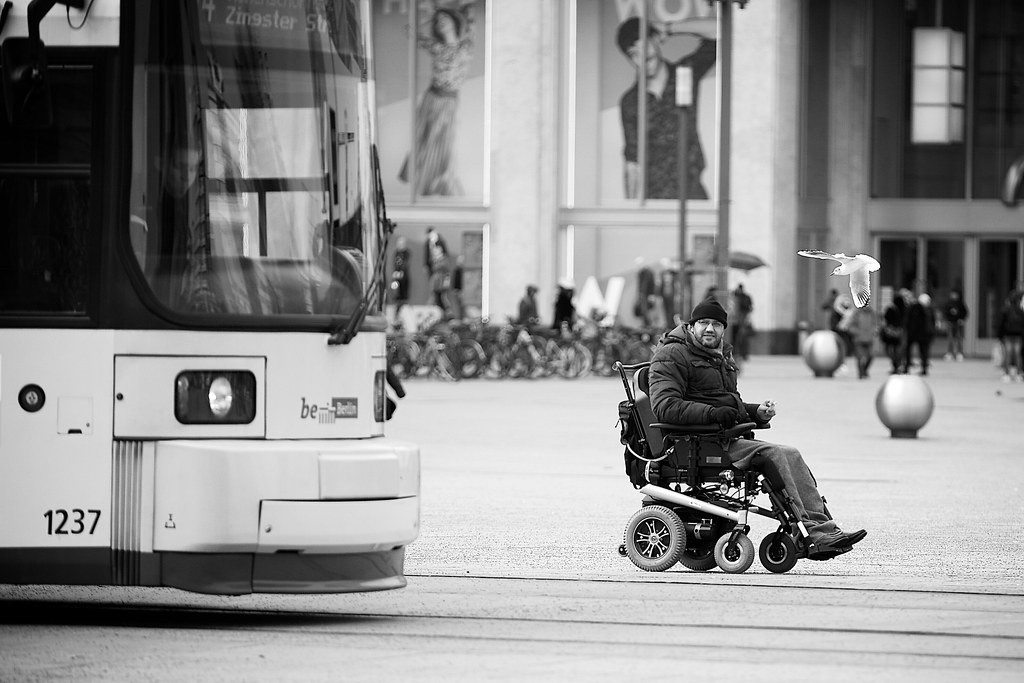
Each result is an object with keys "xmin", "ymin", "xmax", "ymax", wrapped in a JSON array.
[{"xmin": 795, "ymin": 250, "xmax": 881, "ymax": 308}]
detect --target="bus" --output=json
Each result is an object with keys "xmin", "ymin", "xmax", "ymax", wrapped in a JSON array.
[{"xmin": 0, "ymin": 0, "xmax": 420, "ymax": 595}]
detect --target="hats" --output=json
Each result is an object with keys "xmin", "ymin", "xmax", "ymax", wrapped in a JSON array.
[{"xmin": 688, "ymin": 296, "xmax": 728, "ymax": 330}]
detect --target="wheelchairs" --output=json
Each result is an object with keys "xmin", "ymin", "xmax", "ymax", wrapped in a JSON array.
[{"xmin": 612, "ymin": 362, "xmax": 853, "ymax": 575}]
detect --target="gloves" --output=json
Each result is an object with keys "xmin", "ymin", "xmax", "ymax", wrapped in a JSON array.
[{"xmin": 710, "ymin": 406, "xmax": 738, "ymax": 429}]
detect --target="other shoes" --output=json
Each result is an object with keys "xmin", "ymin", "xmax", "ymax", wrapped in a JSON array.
[
  {"xmin": 837, "ymin": 529, "xmax": 867, "ymax": 547},
  {"xmin": 815, "ymin": 534, "xmax": 848, "ymax": 547}
]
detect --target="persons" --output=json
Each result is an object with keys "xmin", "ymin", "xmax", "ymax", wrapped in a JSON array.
[
  {"xmin": 648, "ymin": 296, "xmax": 867, "ymax": 559},
  {"xmin": 822, "ymin": 289, "xmax": 1024, "ymax": 384},
  {"xmin": 398, "ymin": 0, "xmax": 478, "ymax": 196},
  {"xmin": 552, "ymin": 284, "xmax": 573, "ymax": 330},
  {"xmin": 615, "ymin": 16, "xmax": 717, "ymax": 199},
  {"xmin": 520, "ymin": 287, "xmax": 539, "ymax": 323},
  {"xmin": 704, "ymin": 284, "xmax": 753, "ymax": 359}
]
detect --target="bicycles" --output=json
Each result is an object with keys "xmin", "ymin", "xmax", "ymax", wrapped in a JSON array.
[{"xmin": 388, "ymin": 319, "xmax": 656, "ymax": 381}]
